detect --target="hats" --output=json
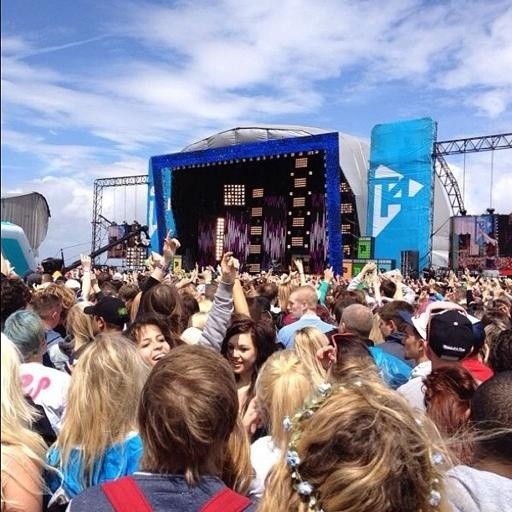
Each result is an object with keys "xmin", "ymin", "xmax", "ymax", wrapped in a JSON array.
[
  {"xmin": 398, "ymin": 301, "xmax": 485, "ymax": 361},
  {"xmin": 83, "ymin": 298, "xmax": 130, "ymax": 324}
]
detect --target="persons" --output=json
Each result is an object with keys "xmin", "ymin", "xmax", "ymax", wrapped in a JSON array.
[
  {"xmin": 443, "ymin": 370, "xmax": 512, "ymax": 512},
  {"xmin": 42, "ymin": 334, "xmax": 149, "ymax": 512},
  {"xmin": 1, "ymin": 230, "xmax": 511, "ymax": 439},
  {"xmin": 2, "ymin": 334, "xmax": 48, "ymax": 511},
  {"xmin": 422, "ymin": 365, "xmax": 475, "ymax": 506},
  {"xmin": 264, "ymin": 379, "xmax": 442, "ymax": 510},
  {"xmin": 243, "ymin": 350, "xmax": 310, "ymax": 512},
  {"xmin": 65, "ymin": 346, "xmax": 258, "ymax": 512}
]
